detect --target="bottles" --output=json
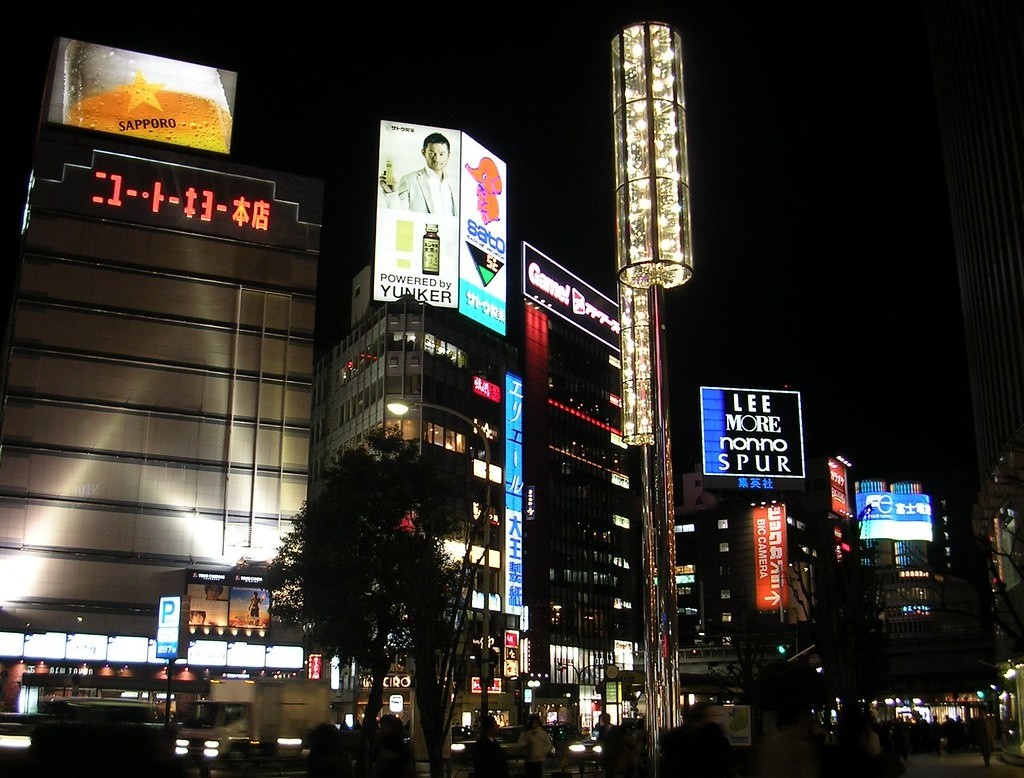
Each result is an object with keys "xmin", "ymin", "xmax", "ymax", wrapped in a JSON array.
[
  {"xmin": 422, "ymin": 223, "xmax": 440, "ymax": 276},
  {"xmin": 385, "ymin": 158, "xmax": 394, "ymax": 185}
]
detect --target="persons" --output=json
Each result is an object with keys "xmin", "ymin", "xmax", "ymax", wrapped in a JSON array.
[
  {"xmin": 305, "ymin": 701, "xmax": 1019, "ymax": 778},
  {"xmin": 379, "ymin": 132, "xmax": 458, "ymax": 218},
  {"xmin": 188, "ymin": 583, "xmax": 264, "ymax": 628}
]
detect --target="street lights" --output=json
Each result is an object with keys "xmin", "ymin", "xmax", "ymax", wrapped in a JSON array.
[
  {"xmin": 559, "ymin": 662, "xmax": 601, "ymax": 736},
  {"xmin": 387, "ymin": 399, "xmax": 490, "ymax": 716}
]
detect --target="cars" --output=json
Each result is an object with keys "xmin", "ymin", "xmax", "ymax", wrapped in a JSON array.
[
  {"xmin": 403, "ymin": 723, "xmax": 482, "ymax": 755},
  {"xmin": 541, "ymin": 722, "xmax": 602, "ymax": 761}
]
